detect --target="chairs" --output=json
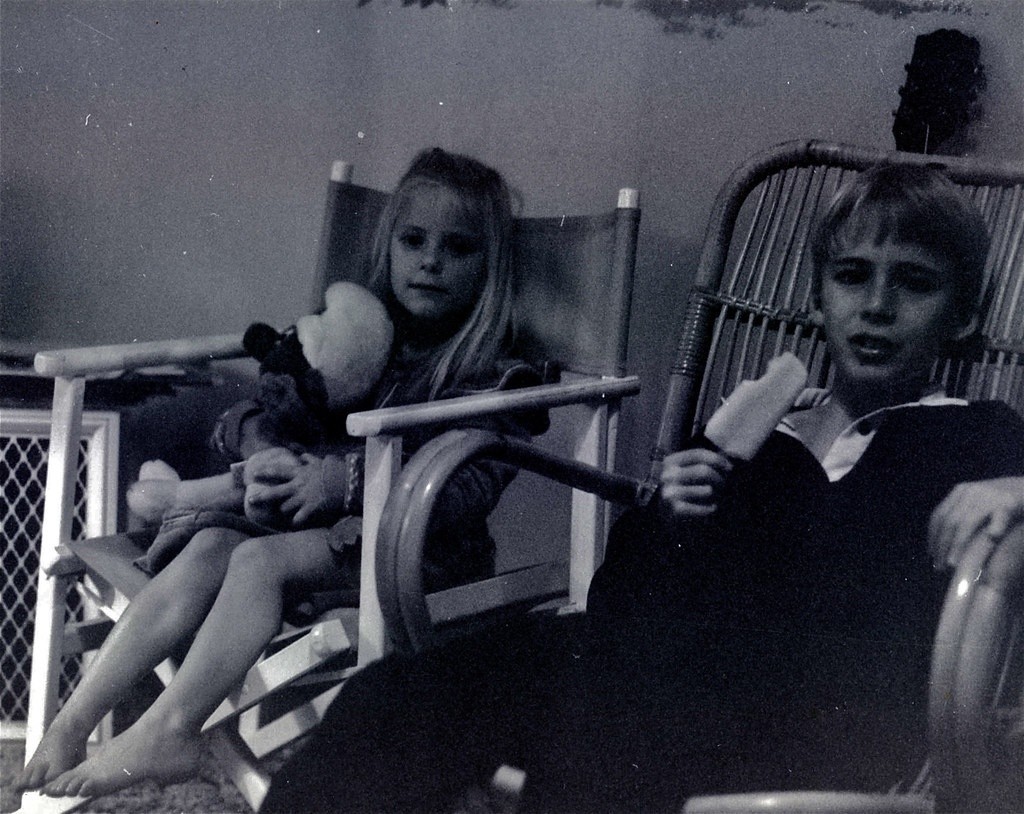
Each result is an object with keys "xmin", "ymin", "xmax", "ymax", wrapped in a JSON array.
[
  {"xmin": 6, "ymin": 159, "xmax": 642, "ymax": 814},
  {"xmin": 374, "ymin": 135, "xmax": 1024, "ymax": 814}
]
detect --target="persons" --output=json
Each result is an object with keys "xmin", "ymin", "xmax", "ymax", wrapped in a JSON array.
[
  {"xmin": 257, "ymin": 163, "xmax": 1024, "ymax": 814},
  {"xmin": 12, "ymin": 148, "xmax": 551, "ymax": 797}
]
